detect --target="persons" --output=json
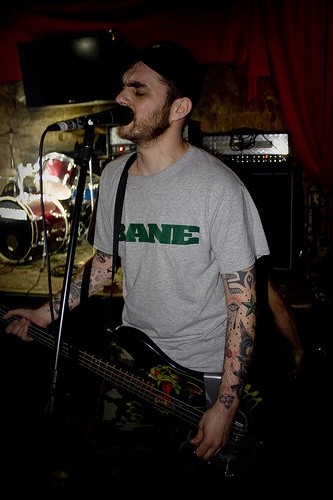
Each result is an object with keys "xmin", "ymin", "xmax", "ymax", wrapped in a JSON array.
[
  {"xmin": 1, "ymin": 40, "xmax": 269, "ymax": 478},
  {"xmin": 256, "ymin": 253, "xmax": 305, "ymax": 368}
]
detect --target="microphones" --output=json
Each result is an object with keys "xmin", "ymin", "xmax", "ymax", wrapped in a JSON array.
[{"xmin": 46, "ymin": 106, "xmax": 135, "ymax": 131}]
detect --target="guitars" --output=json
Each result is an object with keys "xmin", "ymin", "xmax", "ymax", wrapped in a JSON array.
[{"xmin": 0, "ymin": 303, "xmax": 265, "ymax": 482}]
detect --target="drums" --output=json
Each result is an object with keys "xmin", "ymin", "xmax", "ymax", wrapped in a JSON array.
[
  {"xmin": 31, "ymin": 153, "xmax": 80, "ymax": 201},
  {"xmin": 0, "ymin": 192, "xmax": 70, "ymax": 263}
]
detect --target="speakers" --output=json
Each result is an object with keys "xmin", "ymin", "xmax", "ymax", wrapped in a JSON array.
[{"xmin": 235, "ymin": 170, "xmax": 293, "ymax": 272}]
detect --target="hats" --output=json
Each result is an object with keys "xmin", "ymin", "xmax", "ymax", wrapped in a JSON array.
[{"xmin": 122, "ymin": 38, "xmax": 205, "ymax": 95}]
